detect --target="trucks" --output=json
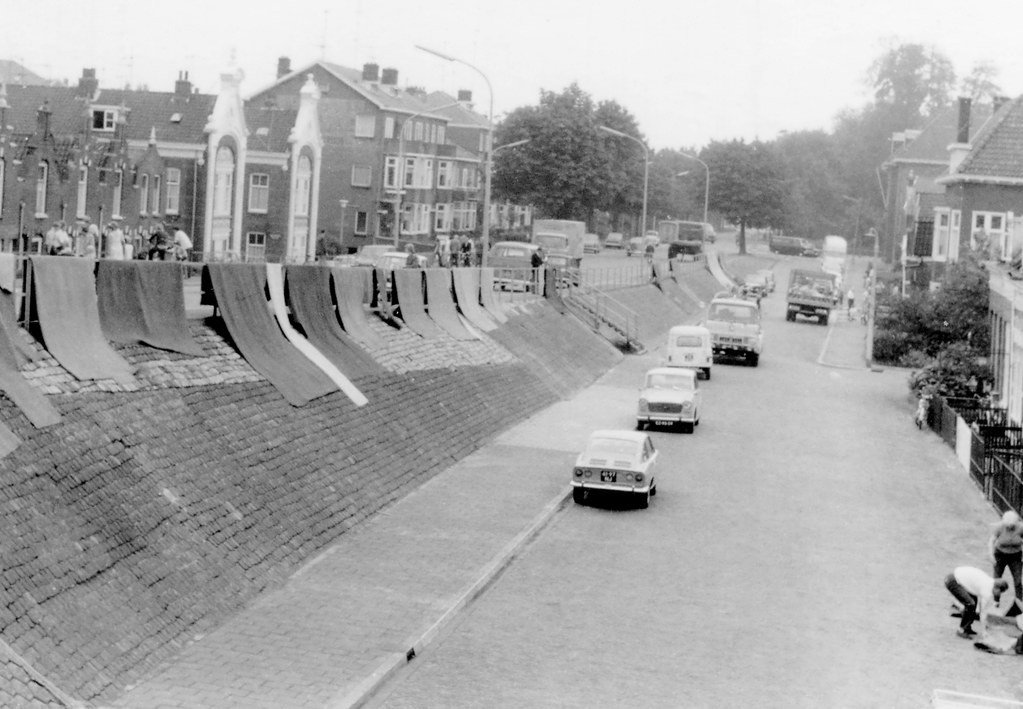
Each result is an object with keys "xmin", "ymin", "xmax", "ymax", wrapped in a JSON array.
[
  {"xmin": 785, "ymin": 262, "xmax": 836, "ymax": 326},
  {"xmin": 531, "ymin": 219, "xmax": 586, "ymax": 268},
  {"xmin": 706, "ymin": 291, "xmax": 764, "ymax": 367}
]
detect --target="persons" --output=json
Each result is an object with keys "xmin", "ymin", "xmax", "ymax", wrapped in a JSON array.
[
  {"xmin": 46, "ymin": 220, "xmax": 73, "ymax": 257},
  {"xmin": 449, "ymin": 234, "xmax": 474, "ymax": 267},
  {"xmin": 403, "ymin": 243, "xmax": 419, "ymax": 268},
  {"xmin": 172, "ymin": 225, "xmax": 192, "ymax": 259},
  {"xmin": 78, "ymin": 220, "xmax": 99, "ymax": 258},
  {"xmin": 316, "ymin": 229, "xmax": 328, "ymax": 256},
  {"xmin": 645, "ymin": 241, "xmax": 654, "ymax": 264},
  {"xmin": 532, "ymin": 248, "xmax": 543, "ymax": 292},
  {"xmin": 105, "ymin": 221, "xmax": 125, "ymax": 260},
  {"xmin": 172, "ymin": 240, "xmax": 188, "ymax": 278},
  {"xmin": 989, "ymin": 511, "xmax": 1023, "ymax": 608},
  {"xmin": 944, "ymin": 567, "xmax": 1009, "ymax": 640},
  {"xmin": 147, "ymin": 223, "xmax": 167, "ymax": 261}
]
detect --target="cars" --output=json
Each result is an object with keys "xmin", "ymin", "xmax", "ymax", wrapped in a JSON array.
[
  {"xmin": 637, "ymin": 368, "xmax": 700, "ymax": 434},
  {"xmin": 652, "ymin": 241, "xmax": 707, "ymax": 282},
  {"xmin": 665, "ymin": 325, "xmax": 713, "ymax": 379},
  {"xmin": 488, "ymin": 240, "xmax": 579, "ymax": 294},
  {"xmin": 605, "ymin": 232, "xmax": 625, "ymax": 249},
  {"xmin": 584, "ymin": 233, "xmax": 602, "ymax": 253},
  {"xmin": 570, "ymin": 429, "xmax": 660, "ymax": 507},
  {"xmin": 736, "ymin": 270, "xmax": 775, "ymax": 306},
  {"xmin": 645, "ymin": 231, "xmax": 660, "ymax": 246},
  {"xmin": 332, "ymin": 242, "xmax": 428, "ymax": 298},
  {"xmin": 627, "ymin": 237, "xmax": 642, "ymax": 256},
  {"xmin": 821, "ymin": 261, "xmax": 843, "ymax": 285}
]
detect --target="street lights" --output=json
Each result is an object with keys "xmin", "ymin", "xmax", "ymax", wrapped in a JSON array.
[
  {"xmin": 864, "ymin": 226, "xmax": 878, "ymax": 367},
  {"xmin": 677, "ymin": 151, "xmax": 710, "ymax": 219},
  {"xmin": 190, "ymin": 158, "xmax": 204, "ymax": 243},
  {"xmin": 601, "ymin": 125, "xmax": 649, "ymax": 236},
  {"xmin": 842, "ymin": 195, "xmax": 863, "ymax": 263},
  {"xmin": 394, "ymin": 100, "xmax": 476, "ymax": 245},
  {"xmin": 416, "ymin": 45, "xmax": 530, "ymax": 298}
]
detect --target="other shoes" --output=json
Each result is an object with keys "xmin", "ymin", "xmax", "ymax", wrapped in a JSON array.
[{"xmin": 957, "ymin": 629, "xmax": 970, "ymax": 639}]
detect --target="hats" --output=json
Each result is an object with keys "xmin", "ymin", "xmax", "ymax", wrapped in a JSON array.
[{"xmin": 996, "ymin": 579, "xmax": 1008, "ymax": 592}]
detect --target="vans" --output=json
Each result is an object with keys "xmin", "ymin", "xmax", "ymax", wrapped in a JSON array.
[
  {"xmin": 821, "ymin": 235, "xmax": 846, "ymax": 272},
  {"xmin": 658, "ymin": 220, "xmax": 716, "ymax": 244},
  {"xmin": 770, "ymin": 236, "xmax": 819, "ymax": 256}
]
